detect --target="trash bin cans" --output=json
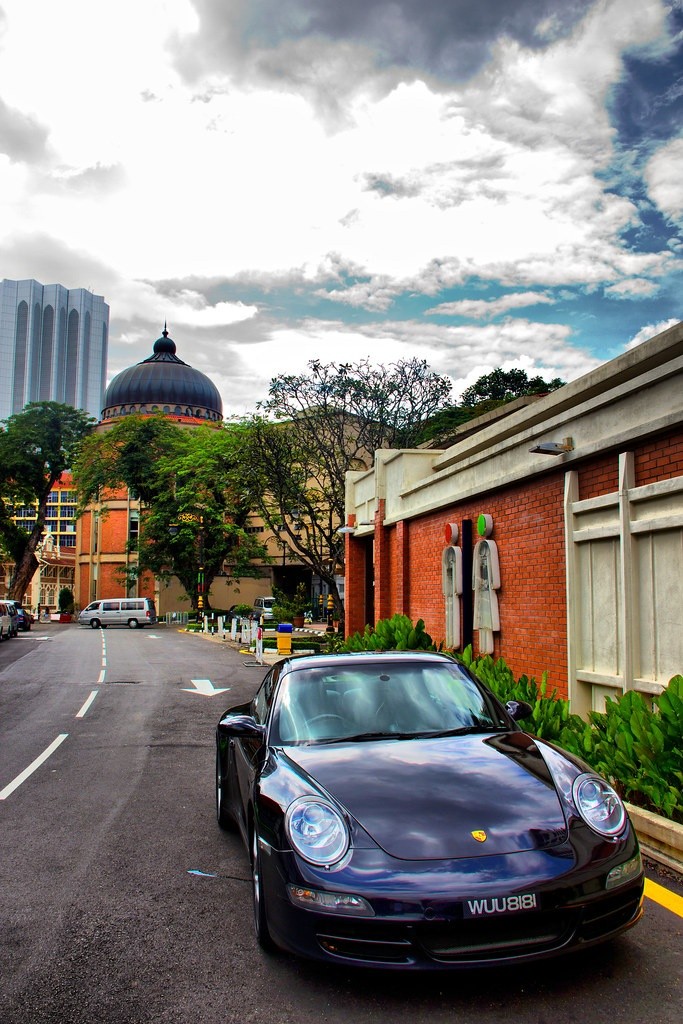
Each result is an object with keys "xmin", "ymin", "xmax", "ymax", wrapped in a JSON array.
[{"xmin": 277, "ymin": 623, "xmax": 293, "ymax": 655}]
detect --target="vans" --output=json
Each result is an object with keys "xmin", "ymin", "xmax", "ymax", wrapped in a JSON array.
[
  {"xmin": 77, "ymin": 598, "xmax": 156, "ymax": 628},
  {"xmin": 253, "ymin": 596, "xmax": 276, "ymax": 620},
  {"xmin": 0, "ymin": 600, "xmax": 20, "ymax": 638}
]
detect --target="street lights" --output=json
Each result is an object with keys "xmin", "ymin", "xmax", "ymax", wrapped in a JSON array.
[
  {"xmin": 263, "ymin": 536, "xmax": 285, "ymax": 599},
  {"xmin": 168, "ymin": 505, "xmax": 204, "ymax": 630},
  {"xmin": 294, "ymin": 522, "xmax": 323, "ymax": 622}
]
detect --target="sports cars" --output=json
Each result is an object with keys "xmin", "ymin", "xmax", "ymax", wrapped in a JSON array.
[{"xmin": 217, "ymin": 652, "xmax": 645, "ymax": 971}]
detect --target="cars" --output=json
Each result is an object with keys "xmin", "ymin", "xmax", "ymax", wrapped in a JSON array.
[
  {"xmin": 228, "ymin": 604, "xmax": 257, "ymax": 622},
  {"xmin": 17, "ymin": 609, "xmax": 31, "ymax": 631}
]
